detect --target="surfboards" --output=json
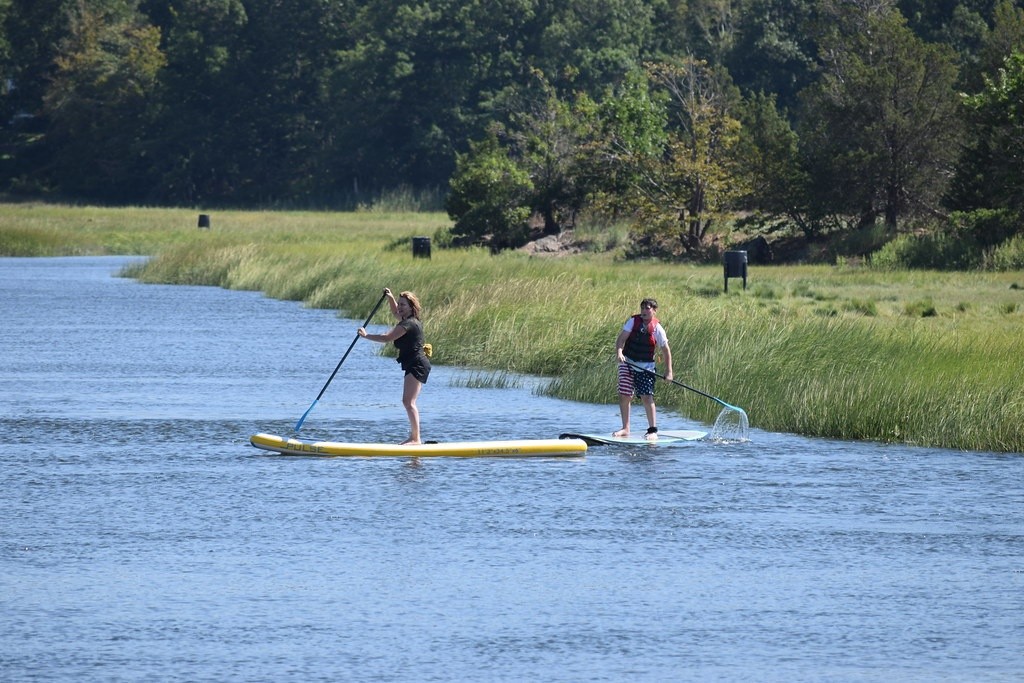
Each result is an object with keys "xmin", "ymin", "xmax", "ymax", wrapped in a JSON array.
[
  {"xmin": 251, "ymin": 432, "xmax": 589, "ymax": 459},
  {"xmin": 557, "ymin": 428, "xmax": 710, "ymax": 448}
]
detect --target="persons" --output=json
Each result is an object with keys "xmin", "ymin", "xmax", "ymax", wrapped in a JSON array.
[
  {"xmin": 612, "ymin": 299, "xmax": 672, "ymax": 439},
  {"xmin": 357, "ymin": 288, "xmax": 432, "ymax": 445}
]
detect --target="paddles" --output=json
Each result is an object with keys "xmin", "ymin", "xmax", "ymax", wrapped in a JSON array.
[
  {"xmin": 297, "ymin": 291, "xmax": 390, "ymax": 429},
  {"xmin": 620, "ymin": 356, "xmax": 746, "ymax": 413}
]
